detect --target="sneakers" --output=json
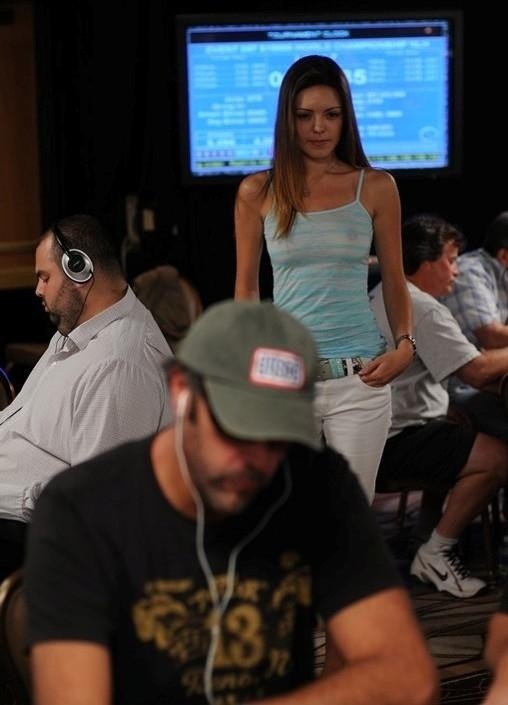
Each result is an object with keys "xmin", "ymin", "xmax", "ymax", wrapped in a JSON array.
[{"xmin": 412, "ymin": 544, "xmax": 487, "ymax": 599}]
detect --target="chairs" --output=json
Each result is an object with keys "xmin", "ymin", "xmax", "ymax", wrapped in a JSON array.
[
  {"xmin": 0, "ymin": 571, "xmax": 30, "ymax": 702},
  {"xmin": 371, "ymin": 471, "xmax": 498, "ymax": 586}
]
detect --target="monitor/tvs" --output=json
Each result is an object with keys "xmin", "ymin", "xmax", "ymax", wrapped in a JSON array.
[{"xmin": 176, "ymin": 8, "xmax": 465, "ymax": 191}]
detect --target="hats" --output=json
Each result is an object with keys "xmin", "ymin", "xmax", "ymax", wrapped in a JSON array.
[{"xmin": 178, "ymin": 302, "xmax": 323, "ymax": 451}]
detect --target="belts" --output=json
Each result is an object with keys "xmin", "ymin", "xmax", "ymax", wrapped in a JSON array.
[{"xmin": 315, "ymin": 356, "xmax": 368, "ymax": 379}]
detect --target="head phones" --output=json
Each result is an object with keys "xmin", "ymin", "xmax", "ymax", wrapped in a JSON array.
[{"xmin": 48, "ymin": 221, "xmax": 94, "ymax": 283}]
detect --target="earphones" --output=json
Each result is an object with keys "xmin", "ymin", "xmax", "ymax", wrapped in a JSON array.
[{"xmin": 177, "ymin": 388, "xmax": 191, "ymax": 419}]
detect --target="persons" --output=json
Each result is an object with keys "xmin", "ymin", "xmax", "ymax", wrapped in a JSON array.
[
  {"xmin": 0, "ymin": 212, "xmax": 175, "ymax": 585},
  {"xmin": 474, "ymin": 586, "xmax": 507, "ymax": 705},
  {"xmin": 131, "ymin": 265, "xmax": 203, "ymax": 353},
  {"xmin": 231, "ymin": 52, "xmax": 422, "ymax": 508},
  {"xmin": 26, "ymin": 297, "xmax": 438, "ymax": 705},
  {"xmin": 367, "ymin": 213, "xmax": 508, "ymax": 598}
]
